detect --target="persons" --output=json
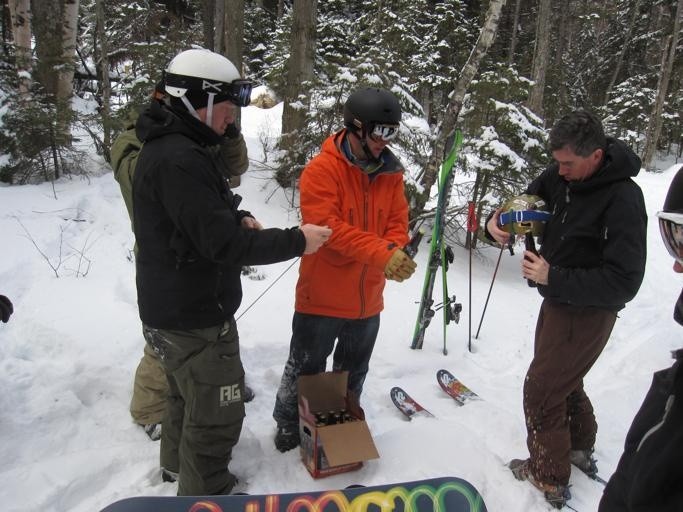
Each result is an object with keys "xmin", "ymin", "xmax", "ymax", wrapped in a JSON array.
[
  {"xmin": 109, "ymin": 81, "xmax": 265, "ymax": 441},
  {"xmin": 131, "ymin": 48, "xmax": 333, "ymax": 497},
  {"xmin": 599, "ymin": 165, "xmax": 683, "ymax": 512},
  {"xmin": 483, "ymin": 109, "xmax": 648, "ymax": 512},
  {"xmin": 272, "ymin": 86, "xmax": 417, "ymax": 453}
]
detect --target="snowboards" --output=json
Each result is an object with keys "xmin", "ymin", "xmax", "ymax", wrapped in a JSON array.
[{"xmin": 99, "ymin": 476, "xmax": 488, "ymax": 512}]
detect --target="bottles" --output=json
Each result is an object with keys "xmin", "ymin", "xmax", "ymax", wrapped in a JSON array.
[
  {"xmin": 403, "ymin": 228, "xmax": 424, "ymax": 258},
  {"xmin": 315, "ymin": 409, "xmax": 352, "ymax": 425},
  {"xmin": 525, "ymin": 229, "xmax": 541, "ymax": 287}
]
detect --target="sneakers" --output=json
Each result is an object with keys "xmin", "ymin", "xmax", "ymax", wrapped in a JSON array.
[
  {"xmin": 510, "ymin": 449, "xmax": 596, "ymax": 498},
  {"xmin": 145, "ymin": 424, "xmax": 162, "ymax": 441},
  {"xmin": 274, "ymin": 423, "xmax": 299, "ymax": 453},
  {"xmin": 161, "ymin": 469, "xmax": 178, "ymax": 482},
  {"xmin": 244, "ymin": 385, "xmax": 255, "ymax": 402},
  {"xmin": 229, "ymin": 477, "xmax": 249, "ymax": 495}
]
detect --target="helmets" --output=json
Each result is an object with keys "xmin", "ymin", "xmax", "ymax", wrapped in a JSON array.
[
  {"xmin": 656, "ymin": 166, "xmax": 683, "ymax": 225},
  {"xmin": 165, "ymin": 48, "xmax": 241, "ymax": 98},
  {"xmin": 344, "ymin": 88, "xmax": 401, "ymax": 126}
]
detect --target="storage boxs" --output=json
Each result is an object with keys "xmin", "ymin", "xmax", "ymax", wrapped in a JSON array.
[{"xmin": 296, "ymin": 370, "xmax": 380, "ymax": 481}]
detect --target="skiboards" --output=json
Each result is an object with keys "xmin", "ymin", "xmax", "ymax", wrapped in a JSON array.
[
  {"xmin": 390, "ymin": 370, "xmax": 609, "ymax": 512},
  {"xmin": 410, "ymin": 127, "xmax": 462, "ymax": 355}
]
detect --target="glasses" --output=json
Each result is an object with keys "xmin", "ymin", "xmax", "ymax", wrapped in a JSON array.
[
  {"xmin": 231, "ymin": 79, "xmax": 252, "ymax": 107},
  {"xmin": 368, "ymin": 122, "xmax": 400, "ymax": 144}
]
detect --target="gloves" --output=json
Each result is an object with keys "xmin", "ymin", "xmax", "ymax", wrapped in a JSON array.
[{"xmin": 384, "ymin": 248, "xmax": 417, "ymax": 282}]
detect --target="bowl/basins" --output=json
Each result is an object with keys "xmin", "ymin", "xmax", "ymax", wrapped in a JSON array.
[{"xmin": 657, "ymin": 211, "xmax": 682, "ymax": 262}]
